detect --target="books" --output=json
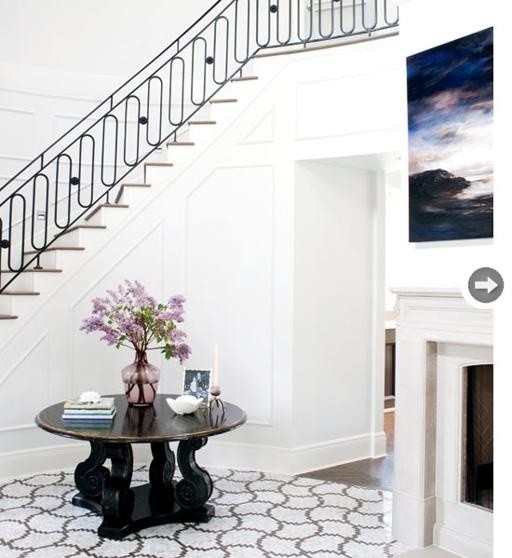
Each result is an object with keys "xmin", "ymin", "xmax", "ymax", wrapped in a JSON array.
[
  {"xmin": 63, "ymin": 404, "xmax": 117, "ymax": 415},
  {"xmin": 63, "ymin": 397, "xmax": 116, "ymax": 410},
  {"xmin": 61, "ymin": 414, "xmax": 117, "ymax": 425},
  {"xmin": 63, "ymin": 420, "xmax": 115, "ymax": 429},
  {"xmin": 62, "ymin": 406, "xmax": 119, "ymax": 420}
]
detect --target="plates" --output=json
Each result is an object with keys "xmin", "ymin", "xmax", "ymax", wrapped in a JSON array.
[{"xmin": 166, "ymin": 394, "xmax": 205, "ymax": 415}]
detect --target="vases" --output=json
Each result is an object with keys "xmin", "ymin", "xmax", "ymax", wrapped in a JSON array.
[{"xmin": 121, "ymin": 350, "xmax": 161, "ymax": 409}]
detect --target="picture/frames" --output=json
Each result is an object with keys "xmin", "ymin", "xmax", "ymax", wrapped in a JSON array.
[{"xmin": 181, "ymin": 367, "xmax": 213, "ymax": 408}]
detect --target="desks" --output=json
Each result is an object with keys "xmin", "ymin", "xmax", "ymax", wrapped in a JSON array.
[{"xmin": 35, "ymin": 393, "xmax": 247, "ymax": 541}]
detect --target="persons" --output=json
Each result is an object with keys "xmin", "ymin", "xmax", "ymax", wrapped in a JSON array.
[
  {"xmin": 196, "ymin": 372, "xmax": 208, "ymax": 392},
  {"xmin": 189, "ymin": 375, "xmax": 197, "ymax": 396}
]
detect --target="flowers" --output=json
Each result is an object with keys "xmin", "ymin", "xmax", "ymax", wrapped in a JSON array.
[{"xmin": 77, "ymin": 278, "xmax": 192, "ymax": 405}]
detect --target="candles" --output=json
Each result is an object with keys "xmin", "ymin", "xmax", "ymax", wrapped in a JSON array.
[{"xmin": 213, "ymin": 343, "xmax": 217, "ymax": 386}]
